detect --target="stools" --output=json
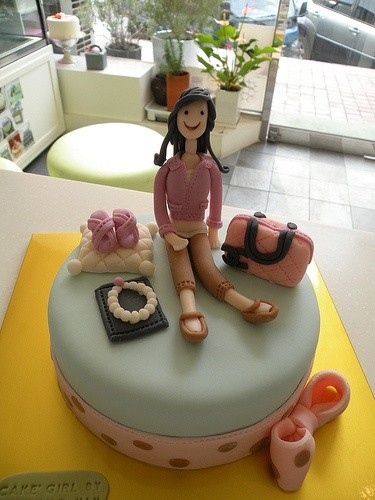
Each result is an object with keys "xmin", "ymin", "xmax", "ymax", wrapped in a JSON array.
[
  {"xmin": 48, "ymin": 213, "xmax": 321, "ymax": 471},
  {"xmin": 46, "ymin": 123, "xmax": 174, "ymax": 193}
]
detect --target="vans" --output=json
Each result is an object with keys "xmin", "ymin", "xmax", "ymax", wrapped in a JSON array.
[{"xmin": 113, "ymin": 1, "xmax": 301, "ymax": 59}]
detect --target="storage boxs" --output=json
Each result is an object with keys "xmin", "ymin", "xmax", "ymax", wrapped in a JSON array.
[{"xmin": 84, "ymin": 44, "xmax": 107, "ymax": 70}]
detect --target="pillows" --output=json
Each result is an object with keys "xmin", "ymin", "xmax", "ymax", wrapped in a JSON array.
[{"xmin": 67, "ymin": 223, "xmax": 159, "ymax": 277}]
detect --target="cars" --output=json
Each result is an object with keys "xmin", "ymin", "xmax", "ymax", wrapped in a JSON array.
[{"xmin": 295, "ymin": 0, "xmax": 375, "ymax": 68}]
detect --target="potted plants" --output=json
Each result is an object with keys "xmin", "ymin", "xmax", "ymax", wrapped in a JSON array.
[{"xmin": 78, "ymin": 0, "xmax": 280, "ymax": 130}]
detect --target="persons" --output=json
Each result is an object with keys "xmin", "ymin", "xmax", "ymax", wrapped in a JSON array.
[
  {"xmin": 153, "ymin": 87, "xmax": 280, "ymax": 342},
  {"xmin": 213, "ymin": 2, "xmax": 239, "ymax": 49}
]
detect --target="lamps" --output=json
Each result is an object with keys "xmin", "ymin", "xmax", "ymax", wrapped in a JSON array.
[{"xmin": 47, "ymin": 15, "xmax": 81, "ymax": 65}]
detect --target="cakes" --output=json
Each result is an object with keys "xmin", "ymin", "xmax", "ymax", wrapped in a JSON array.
[
  {"xmin": 46, "ymin": 12, "xmax": 80, "ymax": 39},
  {"xmin": 48, "ymin": 87, "xmax": 350, "ymax": 492}
]
